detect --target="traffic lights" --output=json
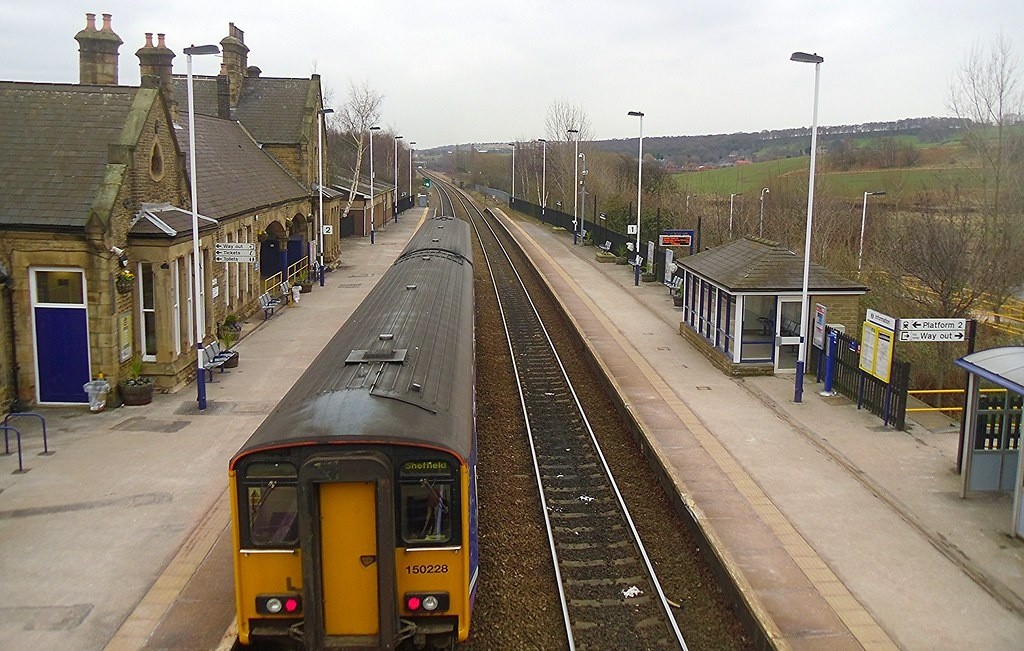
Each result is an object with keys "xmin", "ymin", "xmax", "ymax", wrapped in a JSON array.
[{"xmin": 426, "ymin": 178, "xmax": 430, "ymax": 187}]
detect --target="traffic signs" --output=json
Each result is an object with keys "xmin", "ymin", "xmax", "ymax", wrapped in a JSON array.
[
  {"xmin": 898, "ymin": 319, "xmax": 966, "ymax": 341},
  {"xmin": 215, "ymin": 243, "xmax": 256, "ymax": 262}
]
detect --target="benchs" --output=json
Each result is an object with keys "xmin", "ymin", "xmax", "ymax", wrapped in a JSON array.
[
  {"xmin": 758, "ymin": 310, "xmax": 776, "ymax": 336},
  {"xmin": 259, "ymin": 292, "xmax": 282, "ymax": 320},
  {"xmin": 577, "ymin": 229, "xmax": 587, "ymax": 239},
  {"xmin": 280, "ymin": 280, "xmax": 293, "ymax": 306},
  {"xmin": 665, "ymin": 276, "xmax": 683, "ymax": 295},
  {"xmin": 627, "ymin": 255, "xmax": 643, "ymax": 271},
  {"xmin": 778, "ymin": 319, "xmax": 801, "ymax": 352},
  {"xmin": 200, "ymin": 340, "xmax": 236, "ymax": 383},
  {"xmin": 599, "ymin": 240, "xmax": 611, "ymax": 252}
]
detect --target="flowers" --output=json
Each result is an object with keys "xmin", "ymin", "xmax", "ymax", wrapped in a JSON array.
[{"xmin": 117, "ymin": 269, "xmax": 135, "ymax": 283}]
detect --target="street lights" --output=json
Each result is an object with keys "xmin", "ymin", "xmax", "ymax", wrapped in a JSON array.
[
  {"xmin": 182, "ymin": 44, "xmax": 221, "ymax": 411},
  {"xmin": 538, "ymin": 139, "xmax": 546, "ymax": 224},
  {"xmin": 509, "ymin": 143, "xmax": 515, "ymax": 211},
  {"xmin": 317, "ymin": 109, "xmax": 335, "ymax": 287},
  {"xmin": 628, "ymin": 111, "xmax": 644, "ymax": 286},
  {"xmin": 567, "ymin": 129, "xmax": 578, "ymax": 245},
  {"xmin": 370, "ymin": 127, "xmax": 382, "ymax": 244},
  {"xmin": 857, "ymin": 191, "xmax": 886, "ymax": 281},
  {"xmin": 394, "ymin": 136, "xmax": 403, "ymax": 223},
  {"xmin": 410, "ymin": 142, "xmax": 416, "ymax": 209},
  {"xmin": 790, "ymin": 52, "xmax": 824, "ymax": 403}
]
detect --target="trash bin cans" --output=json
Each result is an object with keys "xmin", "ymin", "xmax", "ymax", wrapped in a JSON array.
[{"xmin": 83, "ymin": 380, "xmax": 111, "ymax": 413}]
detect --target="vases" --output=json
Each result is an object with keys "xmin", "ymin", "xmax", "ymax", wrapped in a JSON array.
[{"xmin": 117, "ymin": 280, "xmax": 134, "ymax": 294}]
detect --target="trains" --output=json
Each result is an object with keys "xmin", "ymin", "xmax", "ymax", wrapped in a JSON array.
[{"xmin": 226, "ymin": 216, "xmax": 476, "ymax": 651}]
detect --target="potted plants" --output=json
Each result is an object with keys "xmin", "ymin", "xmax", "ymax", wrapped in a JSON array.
[
  {"xmin": 218, "ymin": 330, "xmax": 239, "ymax": 368},
  {"xmin": 674, "ymin": 283, "xmax": 685, "ymax": 307},
  {"xmin": 640, "ymin": 261, "xmax": 657, "ymax": 282},
  {"xmin": 584, "ymin": 230, "xmax": 594, "ymax": 246},
  {"xmin": 117, "ymin": 348, "xmax": 154, "ymax": 405},
  {"xmin": 294, "ymin": 269, "xmax": 314, "ymax": 292},
  {"xmin": 615, "ymin": 243, "xmax": 627, "ymax": 264}
]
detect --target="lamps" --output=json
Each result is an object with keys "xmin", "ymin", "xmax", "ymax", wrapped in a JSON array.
[{"xmin": 108, "ymin": 246, "xmax": 125, "ymax": 260}]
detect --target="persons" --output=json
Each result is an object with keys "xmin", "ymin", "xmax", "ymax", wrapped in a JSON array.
[{"xmin": 406, "ymin": 487, "xmax": 451, "ymax": 539}]
